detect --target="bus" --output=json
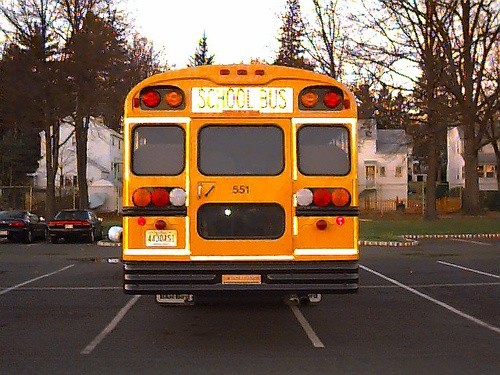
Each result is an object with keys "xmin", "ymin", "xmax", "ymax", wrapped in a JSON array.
[{"xmin": 119, "ymin": 64, "xmax": 360, "ymax": 309}]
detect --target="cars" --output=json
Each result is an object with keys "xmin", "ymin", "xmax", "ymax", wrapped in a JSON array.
[
  {"xmin": 47, "ymin": 210, "xmax": 103, "ymax": 243},
  {"xmin": 0, "ymin": 209, "xmax": 48, "ymax": 245}
]
